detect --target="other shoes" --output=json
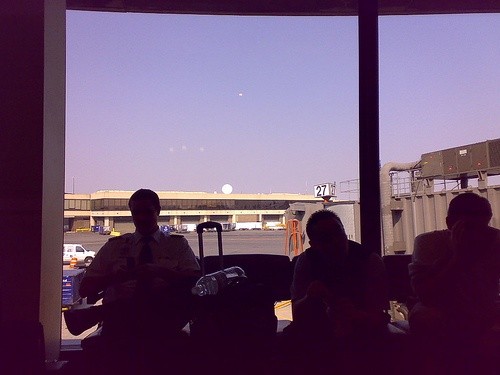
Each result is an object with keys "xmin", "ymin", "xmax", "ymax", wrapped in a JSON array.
[{"xmin": 64, "ymin": 308, "xmax": 102, "ymax": 336}]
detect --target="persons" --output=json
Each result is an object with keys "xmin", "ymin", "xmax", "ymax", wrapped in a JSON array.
[
  {"xmin": 282, "ymin": 209, "xmax": 392, "ymax": 375},
  {"xmin": 64, "ymin": 189, "xmax": 200, "ymax": 375},
  {"xmin": 410, "ymin": 193, "xmax": 500, "ymax": 375}
]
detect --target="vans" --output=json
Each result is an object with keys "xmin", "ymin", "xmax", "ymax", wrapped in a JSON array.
[{"xmin": 63, "ymin": 243, "xmax": 96, "ymax": 265}]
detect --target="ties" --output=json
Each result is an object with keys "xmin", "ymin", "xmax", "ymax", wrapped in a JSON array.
[{"xmin": 136, "ymin": 242, "xmax": 152, "ymax": 261}]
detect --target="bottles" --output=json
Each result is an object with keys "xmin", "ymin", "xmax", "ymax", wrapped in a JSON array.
[{"xmin": 191, "ymin": 266, "xmax": 244, "ymax": 297}]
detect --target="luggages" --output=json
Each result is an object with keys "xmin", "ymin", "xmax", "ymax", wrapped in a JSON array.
[{"xmin": 190, "ymin": 220, "xmax": 276, "ymax": 375}]
